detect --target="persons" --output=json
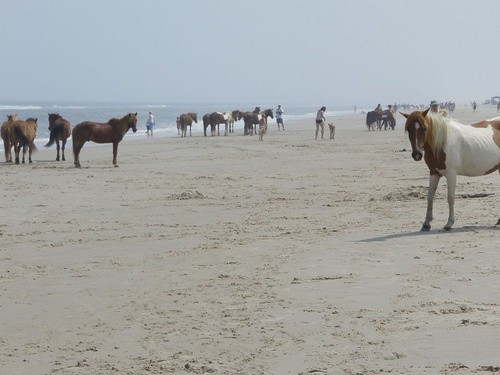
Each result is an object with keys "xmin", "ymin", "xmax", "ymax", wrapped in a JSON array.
[
  {"xmin": 146, "ymin": 112, "xmax": 156, "ymax": 136},
  {"xmin": 314, "ymin": 106, "xmax": 327, "ymax": 140},
  {"xmin": 176, "ymin": 117, "xmax": 182, "ymax": 135},
  {"xmin": 375, "ymin": 104, "xmax": 383, "ymax": 130},
  {"xmin": 275, "ymin": 104, "xmax": 285, "ymax": 132},
  {"xmin": 384, "ymin": 105, "xmax": 394, "ymax": 130},
  {"xmin": 355, "ymin": 99, "xmax": 500, "ymax": 118},
  {"xmin": 328, "ymin": 122, "xmax": 336, "ymax": 139},
  {"xmin": 259, "ymin": 114, "xmax": 266, "ymax": 141}
]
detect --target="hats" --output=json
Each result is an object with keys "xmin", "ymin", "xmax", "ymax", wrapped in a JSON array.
[{"xmin": 430, "ymin": 100, "xmax": 438, "ymax": 106}]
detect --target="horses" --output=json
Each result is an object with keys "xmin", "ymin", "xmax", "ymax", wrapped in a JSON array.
[
  {"xmin": 1, "ymin": 111, "xmax": 139, "ymax": 169},
  {"xmin": 398, "ymin": 106, "xmax": 500, "ymax": 234},
  {"xmin": 180, "ymin": 106, "xmax": 274, "ymax": 138},
  {"xmin": 366, "ymin": 109, "xmax": 397, "ymax": 132}
]
